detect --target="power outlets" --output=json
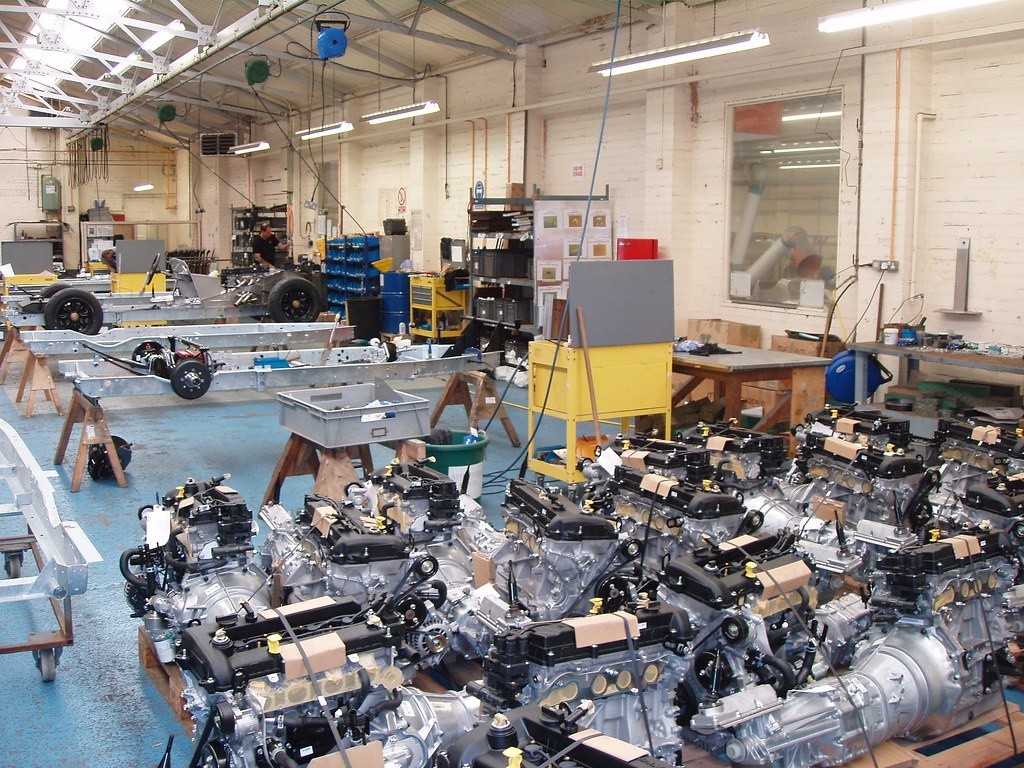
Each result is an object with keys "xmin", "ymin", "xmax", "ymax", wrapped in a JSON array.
[{"xmin": 872, "ymin": 260, "xmax": 899, "ymax": 271}]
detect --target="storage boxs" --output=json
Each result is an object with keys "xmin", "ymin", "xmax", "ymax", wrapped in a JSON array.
[
  {"xmin": 277, "ymin": 382, "xmax": 430, "ymax": 448},
  {"xmin": 471, "ymin": 248, "xmax": 533, "ymax": 325},
  {"xmin": 688, "ymin": 318, "xmax": 844, "ymax": 422}
]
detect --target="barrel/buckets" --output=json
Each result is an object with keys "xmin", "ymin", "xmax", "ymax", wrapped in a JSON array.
[
  {"xmin": 381, "ymin": 271, "xmax": 411, "ymax": 334},
  {"xmin": 883, "ymin": 328, "xmax": 898, "ymax": 345},
  {"xmin": 422, "ymin": 429, "xmax": 489, "ymax": 503},
  {"xmin": 883, "ymin": 292, "xmax": 924, "ymax": 338}
]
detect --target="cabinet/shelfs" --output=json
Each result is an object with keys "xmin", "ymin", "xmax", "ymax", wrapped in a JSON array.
[
  {"xmin": 844, "ymin": 341, "xmax": 1024, "ymax": 440},
  {"xmin": 469, "ymin": 184, "xmax": 610, "ymax": 338},
  {"xmin": 231, "ymin": 204, "xmax": 287, "ymax": 268},
  {"xmin": 13, "ymin": 220, "xmax": 63, "ymax": 259},
  {"xmin": 324, "ymin": 233, "xmax": 380, "ymax": 319}
]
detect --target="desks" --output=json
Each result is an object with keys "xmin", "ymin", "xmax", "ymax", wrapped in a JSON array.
[{"xmin": 634, "ymin": 344, "xmax": 832, "ymax": 461}]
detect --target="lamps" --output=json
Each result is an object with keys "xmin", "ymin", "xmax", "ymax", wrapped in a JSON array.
[
  {"xmin": 50, "ymin": 96, "xmax": 71, "ymax": 118},
  {"xmin": 85, "ymin": 0, "xmax": 186, "ymax": 93},
  {"xmin": 227, "ymin": 92, "xmax": 271, "ymax": 154},
  {"xmin": 134, "ymin": 131, "xmax": 155, "ymax": 192},
  {"xmin": 586, "ymin": 0, "xmax": 771, "ymax": 77},
  {"xmin": 296, "ymin": 58, "xmax": 354, "ymax": 140},
  {"xmin": 816, "ymin": 0, "xmax": 1007, "ymax": 33},
  {"xmin": 358, "ymin": 29, "xmax": 440, "ymax": 125}
]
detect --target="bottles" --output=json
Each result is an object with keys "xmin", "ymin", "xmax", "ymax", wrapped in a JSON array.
[
  {"xmin": 937, "ymin": 335, "xmax": 947, "ymax": 348},
  {"xmin": 399, "ymin": 322, "xmax": 405, "ymax": 334}
]
